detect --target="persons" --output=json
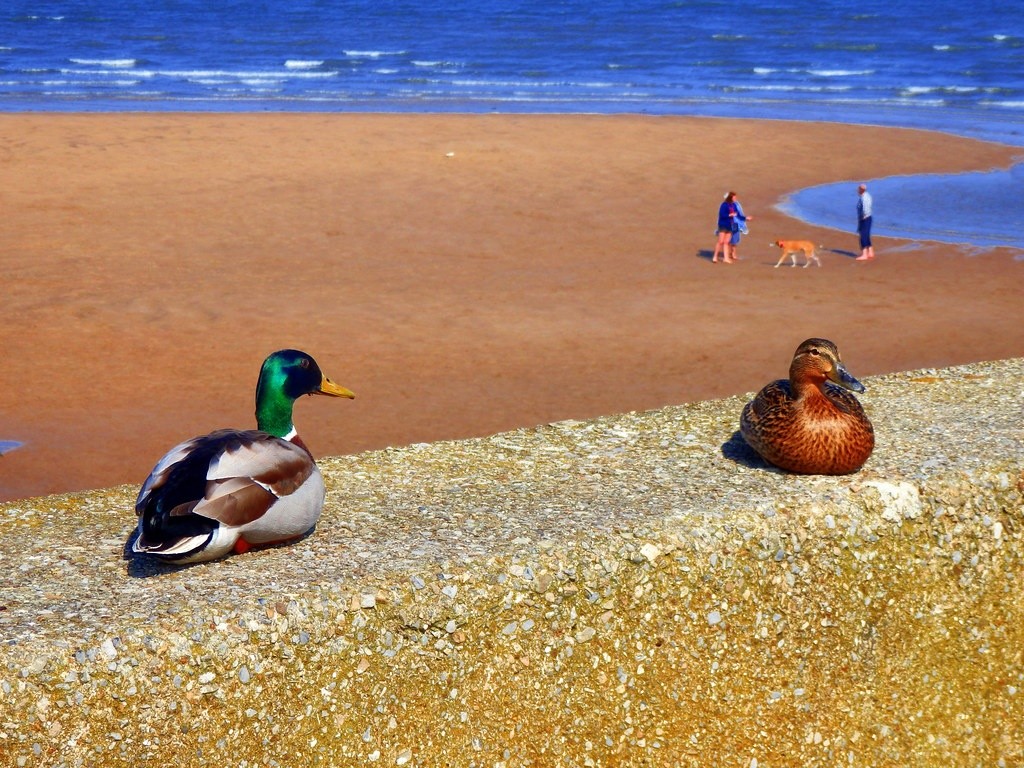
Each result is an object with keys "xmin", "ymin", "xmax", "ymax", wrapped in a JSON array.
[
  {"xmin": 711, "ymin": 192, "xmax": 753, "ymax": 264},
  {"xmin": 853, "ymin": 184, "xmax": 875, "ymax": 260}
]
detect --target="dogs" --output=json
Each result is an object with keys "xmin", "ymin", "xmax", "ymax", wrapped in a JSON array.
[{"xmin": 768, "ymin": 239, "xmax": 825, "ymax": 270}]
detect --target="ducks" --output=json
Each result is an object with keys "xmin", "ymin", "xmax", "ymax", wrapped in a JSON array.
[
  {"xmin": 117, "ymin": 345, "xmax": 358, "ymax": 567},
  {"xmin": 736, "ymin": 335, "xmax": 876, "ymax": 478}
]
